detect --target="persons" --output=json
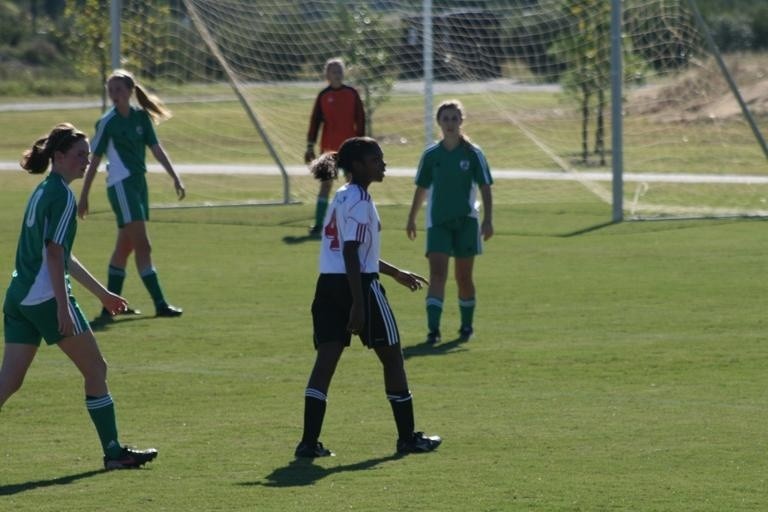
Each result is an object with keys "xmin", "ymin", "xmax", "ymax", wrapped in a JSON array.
[
  {"xmin": 407, "ymin": 97, "xmax": 495, "ymax": 346},
  {"xmin": 0, "ymin": 121, "xmax": 158, "ymax": 469},
  {"xmin": 77, "ymin": 68, "xmax": 187, "ymax": 317},
  {"xmin": 294, "ymin": 137, "xmax": 443, "ymax": 457},
  {"xmin": 304, "ymin": 57, "xmax": 367, "ymax": 234}
]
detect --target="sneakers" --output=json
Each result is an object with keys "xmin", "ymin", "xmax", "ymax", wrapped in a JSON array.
[
  {"xmin": 424, "ymin": 333, "xmax": 441, "ymax": 345},
  {"xmin": 104, "ymin": 445, "xmax": 158, "ymax": 470},
  {"xmin": 101, "ymin": 304, "xmax": 141, "ymax": 316},
  {"xmin": 294, "ymin": 440, "xmax": 331, "ymax": 459},
  {"xmin": 154, "ymin": 304, "xmax": 183, "ymax": 318},
  {"xmin": 394, "ymin": 431, "xmax": 443, "ymax": 455},
  {"xmin": 456, "ymin": 324, "xmax": 474, "ymax": 343}
]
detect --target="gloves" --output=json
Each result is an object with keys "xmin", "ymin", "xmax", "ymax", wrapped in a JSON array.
[{"xmin": 304, "ymin": 143, "xmax": 316, "ymax": 164}]
